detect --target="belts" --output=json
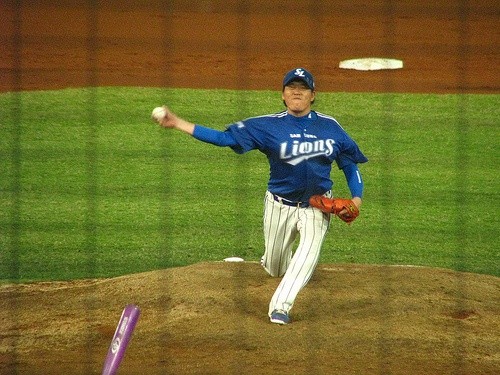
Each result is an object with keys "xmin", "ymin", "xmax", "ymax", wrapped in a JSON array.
[{"xmin": 273, "ymin": 190, "xmax": 332, "ymax": 208}]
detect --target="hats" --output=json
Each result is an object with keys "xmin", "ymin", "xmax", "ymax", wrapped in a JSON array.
[{"xmin": 282, "ymin": 68, "xmax": 315, "ymax": 90}]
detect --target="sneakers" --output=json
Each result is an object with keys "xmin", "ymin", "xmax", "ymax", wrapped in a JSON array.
[{"xmin": 270, "ymin": 310, "xmax": 288, "ymax": 324}]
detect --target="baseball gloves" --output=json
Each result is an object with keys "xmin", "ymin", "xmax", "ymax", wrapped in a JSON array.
[{"xmin": 310, "ymin": 195, "xmax": 361, "ymax": 224}]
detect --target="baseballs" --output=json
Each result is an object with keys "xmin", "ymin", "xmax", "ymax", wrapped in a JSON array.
[
  {"xmin": 99, "ymin": 303, "xmax": 141, "ymax": 375},
  {"xmin": 151, "ymin": 106, "xmax": 165, "ymax": 120}
]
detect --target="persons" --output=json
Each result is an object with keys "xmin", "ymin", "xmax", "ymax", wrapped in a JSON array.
[{"xmin": 152, "ymin": 69, "xmax": 368, "ymax": 324}]
